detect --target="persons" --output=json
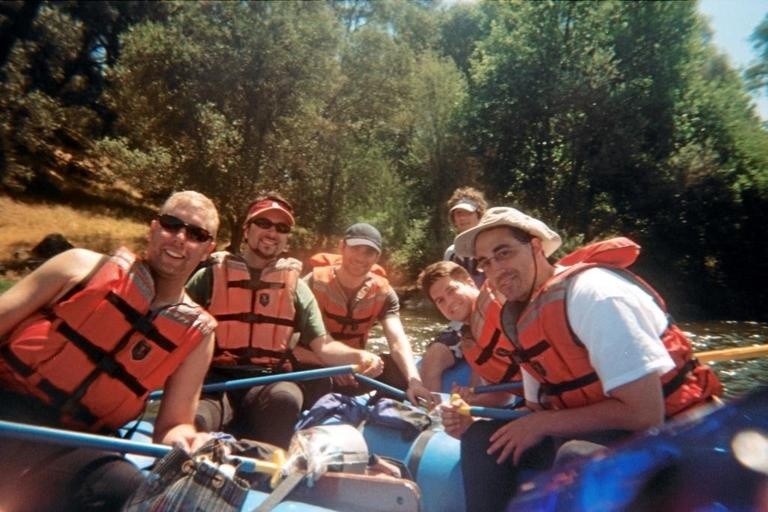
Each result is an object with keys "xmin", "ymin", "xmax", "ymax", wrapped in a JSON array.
[
  {"xmin": 2, "ymin": 188, "xmax": 443, "ymax": 510},
  {"xmin": 411, "ymin": 188, "xmax": 723, "ymax": 511}
]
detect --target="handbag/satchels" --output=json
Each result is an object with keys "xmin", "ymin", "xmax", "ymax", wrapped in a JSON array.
[{"xmin": 119, "ymin": 438, "xmax": 249, "ymax": 512}]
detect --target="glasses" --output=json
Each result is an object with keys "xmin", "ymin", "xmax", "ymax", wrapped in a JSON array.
[
  {"xmin": 249, "ymin": 217, "xmax": 290, "ymax": 234},
  {"xmin": 155, "ymin": 214, "xmax": 214, "ymax": 242}
]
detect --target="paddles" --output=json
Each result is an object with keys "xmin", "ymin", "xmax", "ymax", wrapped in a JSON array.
[{"xmin": 461, "ymin": 346, "xmax": 768, "ymax": 394}]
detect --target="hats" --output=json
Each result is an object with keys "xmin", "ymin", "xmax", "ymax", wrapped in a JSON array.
[
  {"xmin": 244, "ymin": 199, "xmax": 295, "ymax": 227},
  {"xmin": 343, "ymin": 222, "xmax": 381, "ymax": 256},
  {"xmin": 449, "ymin": 199, "xmax": 562, "ymax": 258}
]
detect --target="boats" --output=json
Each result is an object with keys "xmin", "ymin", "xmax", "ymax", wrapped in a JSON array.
[{"xmin": 102, "ymin": 369, "xmax": 768, "ymax": 512}]
